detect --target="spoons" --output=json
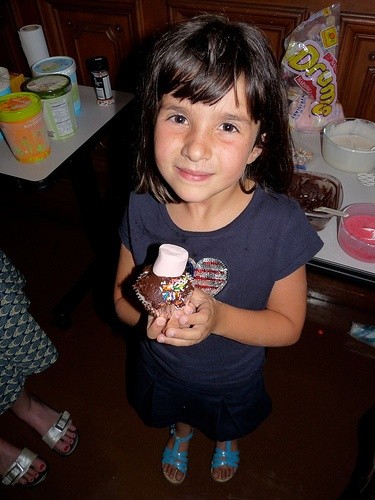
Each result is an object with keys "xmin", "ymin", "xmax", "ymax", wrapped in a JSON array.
[{"xmin": 311, "ymin": 207, "xmax": 349, "ymax": 218}]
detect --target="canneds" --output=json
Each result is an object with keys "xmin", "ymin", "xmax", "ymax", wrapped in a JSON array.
[{"xmin": 93, "ymin": 71, "xmax": 113, "ymax": 101}]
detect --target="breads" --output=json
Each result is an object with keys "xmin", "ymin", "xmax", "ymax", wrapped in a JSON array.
[{"xmin": 132, "ymin": 269, "xmax": 195, "ymax": 318}]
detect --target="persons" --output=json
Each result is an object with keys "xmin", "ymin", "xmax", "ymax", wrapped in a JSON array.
[
  {"xmin": 113, "ymin": 13, "xmax": 324, "ymax": 486},
  {"xmin": 0, "ymin": 255, "xmax": 79, "ymax": 488}
]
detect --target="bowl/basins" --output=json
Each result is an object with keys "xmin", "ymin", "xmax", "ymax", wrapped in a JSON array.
[
  {"xmin": 338, "ymin": 203, "xmax": 375, "ymax": 263},
  {"xmin": 291, "ymin": 172, "xmax": 343, "ymax": 231},
  {"xmin": 322, "ymin": 117, "xmax": 375, "ymax": 172}
]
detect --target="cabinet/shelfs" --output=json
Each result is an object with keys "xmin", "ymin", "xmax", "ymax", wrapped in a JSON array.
[{"xmin": 0, "ymin": 0, "xmax": 374, "ymax": 122}]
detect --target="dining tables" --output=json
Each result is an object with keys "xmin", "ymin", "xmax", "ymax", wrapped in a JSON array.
[
  {"xmin": 289, "ymin": 129, "xmax": 375, "ymax": 346},
  {"xmin": 0, "ymin": 75, "xmax": 137, "ymax": 330}
]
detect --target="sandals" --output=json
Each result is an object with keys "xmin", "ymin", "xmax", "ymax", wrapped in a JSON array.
[
  {"xmin": 11, "ymin": 393, "xmax": 79, "ymax": 456},
  {"xmin": 0, "ymin": 441, "xmax": 48, "ymax": 489},
  {"xmin": 210, "ymin": 440, "xmax": 239, "ymax": 482},
  {"xmin": 161, "ymin": 425, "xmax": 194, "ymax": 484}
]
